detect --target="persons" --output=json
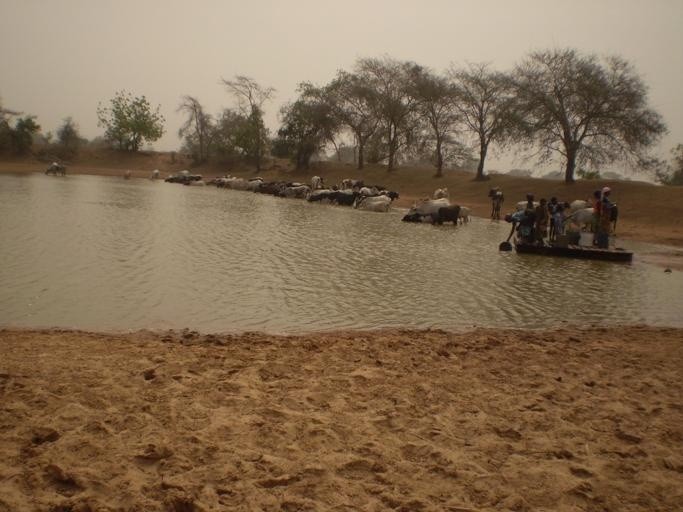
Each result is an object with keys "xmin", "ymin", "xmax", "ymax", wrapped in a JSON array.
[{"xmin": 502, "ymin": 185, "xmax": 617, "ymax": 250}]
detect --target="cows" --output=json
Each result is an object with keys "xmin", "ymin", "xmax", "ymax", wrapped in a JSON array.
[
  {"xmin": 568, "ymin": 199, "xmax": 619, "ymax": 232},
  {"xmin": 45, "ymin": 162, "xmax": 67, "ymax": 176},
  {"xmin": 164, "ymin": 168, "xmax": 400, "ymax": 213},
  {"xmin": 516, "ymin": 200, "xmax": 540, "ymax": 210},
  {"xmin": 401, "ymin": 186, "xmax": 473, "ymax": 227},
  {"xmin": 488, "ymin": 186, "xmax": 503, "ymax": 220},
  {"xmin": 123, "ymin": 169, "xmax": 131, "ymax": 178},
  {"xmin": 149, "ymin": 169, "xmax": 159, "ymax": 179},
  {"xmin": 548, "ymin": 201, "xmax": 570, "ymax": 210}
]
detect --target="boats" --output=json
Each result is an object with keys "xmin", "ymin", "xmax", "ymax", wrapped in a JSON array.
[{"xmin": 514, "ymin": 233, "xmax": 632, "ymax": 262}]
